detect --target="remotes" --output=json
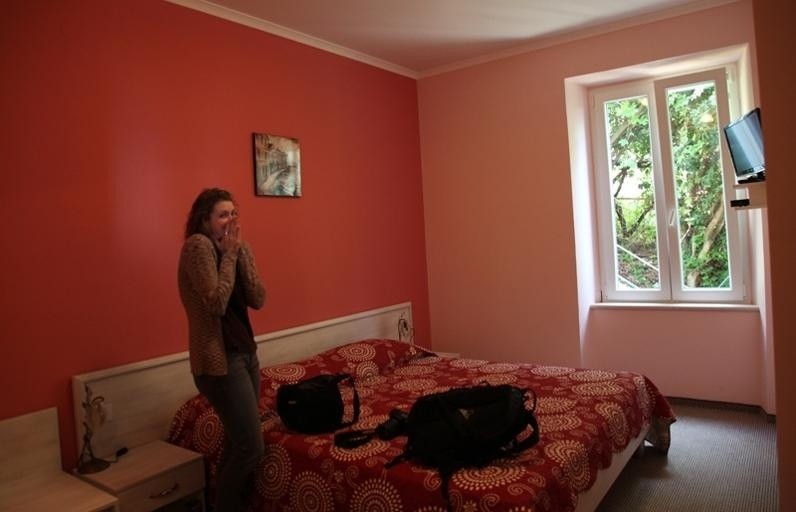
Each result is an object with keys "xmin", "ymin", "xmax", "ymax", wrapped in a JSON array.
[{"xmin": 737, "ymin": 180, "xmax": 751, "ymax": 184}]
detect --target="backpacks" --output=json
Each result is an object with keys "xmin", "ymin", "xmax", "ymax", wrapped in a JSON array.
[{"xmin": 404, "ymin": 380, "xmax": 542, "ymax": 470}]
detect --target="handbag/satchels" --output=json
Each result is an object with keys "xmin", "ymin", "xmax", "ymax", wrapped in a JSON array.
[{"xmin": 275, "ymin": 369, "xmax": 361, "ymax": 435}]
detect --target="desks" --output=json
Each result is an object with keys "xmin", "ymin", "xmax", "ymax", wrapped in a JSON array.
[{"xmin": 0, "ymin": 405, "xmax": 121, "ymax": 512}]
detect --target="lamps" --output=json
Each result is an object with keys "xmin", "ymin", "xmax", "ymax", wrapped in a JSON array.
[{"xmin": 74, "ymin": 382, "xmax": 119, "ymax": 474}]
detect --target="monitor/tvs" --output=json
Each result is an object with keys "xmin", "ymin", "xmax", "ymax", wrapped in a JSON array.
[{"xmin": 722, "ymin": 107, "xmax": 765, "ymax": 181}]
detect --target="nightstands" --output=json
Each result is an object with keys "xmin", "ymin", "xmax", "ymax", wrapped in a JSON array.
[{"xmin": 72, "ymin": 440, "xmax": 210, "ymax": 512}]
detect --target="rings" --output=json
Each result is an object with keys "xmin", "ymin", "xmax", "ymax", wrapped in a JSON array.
[{"xmin": 223, "ymin": 231, "xmax": 228, "ymax": 235}]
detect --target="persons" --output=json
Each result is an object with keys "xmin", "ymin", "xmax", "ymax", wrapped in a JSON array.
[{"xmin": 176, "ymin": 188, "xmax": 266, "ymax": 512}]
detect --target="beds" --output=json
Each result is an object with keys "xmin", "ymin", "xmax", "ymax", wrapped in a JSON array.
[{"xmin": 72, "ymin": 300, "xmax": 676, "ymax": 512}]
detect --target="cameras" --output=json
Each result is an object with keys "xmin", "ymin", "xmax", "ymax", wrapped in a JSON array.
[{"xmin": 375, "ymin": 408, "xmax": 409, "ymax": 441}]
